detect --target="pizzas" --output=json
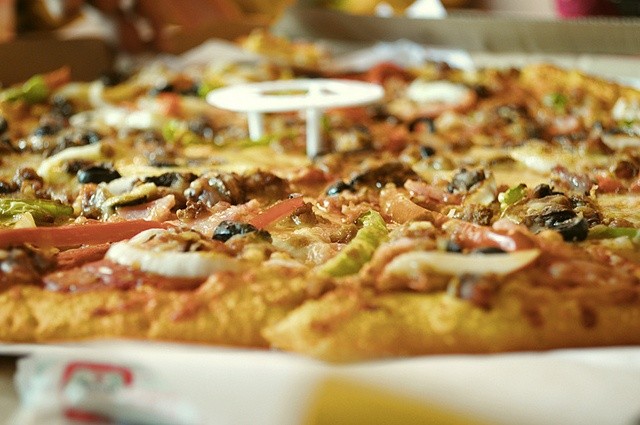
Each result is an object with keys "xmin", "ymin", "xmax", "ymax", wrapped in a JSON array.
[{"xmin": 0, "ymin": 27, "xmax": 636, "ymax": 362}]
